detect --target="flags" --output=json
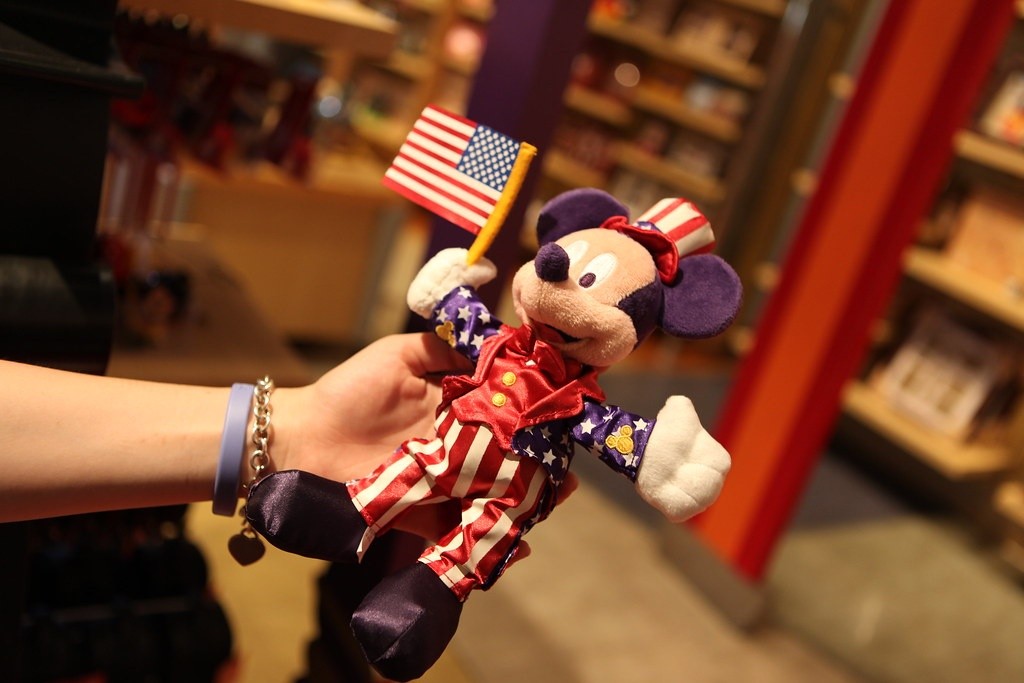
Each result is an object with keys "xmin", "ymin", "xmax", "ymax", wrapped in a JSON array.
[{"xmin": 381, "ymin": 102, "xmax": 521, "ymax": 233}]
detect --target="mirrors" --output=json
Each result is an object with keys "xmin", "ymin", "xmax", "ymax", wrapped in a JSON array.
[{"xmin": 402, "ymin": 3, "xmax": 1013, "ymax": 584}]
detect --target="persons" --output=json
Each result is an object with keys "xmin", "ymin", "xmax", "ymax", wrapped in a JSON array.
[{"xmin": 0, "ymin": 330, "xmax": 578, "ymax": 525}]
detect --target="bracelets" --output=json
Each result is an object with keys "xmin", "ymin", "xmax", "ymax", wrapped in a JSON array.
[{"xmin": 212, "ymin": 375, "xmax": 273, "ymax": 567}]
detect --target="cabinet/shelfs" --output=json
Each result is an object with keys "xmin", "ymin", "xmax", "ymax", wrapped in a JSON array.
[
  {"xmin": 498, "ymin": 0, "xmax": 861, "ymax": 357},
  {"xmin": 820, "ymin": 129, "xmax": 1024, "ymax": 594}
]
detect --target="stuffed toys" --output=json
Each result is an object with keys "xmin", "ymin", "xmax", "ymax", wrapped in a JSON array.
[{"xmin": 242, "ymin": 190, "xmax": 742, "ymax": 683}]
